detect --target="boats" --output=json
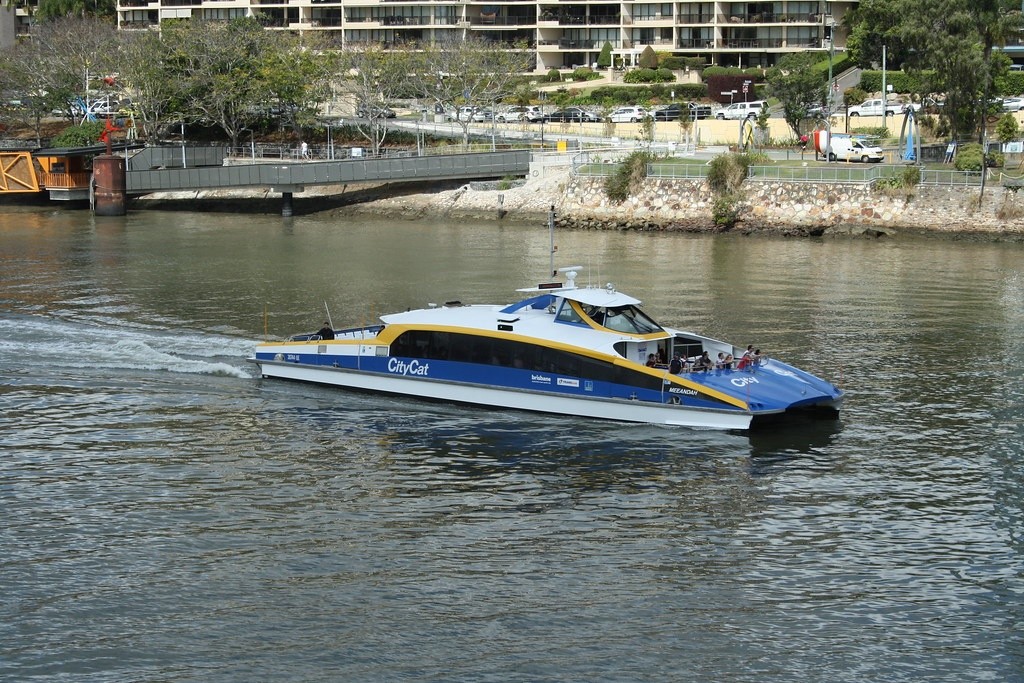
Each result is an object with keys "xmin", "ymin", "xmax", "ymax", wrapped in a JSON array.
[
  {"xmin": 813, "ymin": 130, "xmax": 826, "ymax": 153},
  {"xmin": 248, "ymin": 207, "xmax": 850, "ymax": 432}
]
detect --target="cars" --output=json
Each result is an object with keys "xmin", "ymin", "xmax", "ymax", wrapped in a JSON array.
[
  {"xmin": 905, "ymin": 98, "xmax": 937, "ymax": 115},
  {"xmin": 565, "ymin": 106, "xmax": 603, "ymax": 122},
  {"xmin": 801, "ymin": 105, "xmax": 824, "ymax": 118},
  {"xmin": 655, "ymin": 104, "xmax": 712, "ymax": 122},
  {"xmin": 604, "ymin": 107, "xmax": 647, "ymax": 123},
  {"xmin": 355, "ymin": 101, "xmax": 396, "ymax": 118},
  {"xmin": 46, "ymin": 92, "xmax": 310, "ymax": 119},
  {"xmin": 986, "ymin": 94, "xmax": 1024, "ymax": 111},
  {"xmin": 539, "ymin": 108, "xmax": 587, "ymax": 122},
  {"xmin": 495, "ymin": 105, "xmax": 543, "ymax": 123},
  {"xmin": 448, "ymin": 105, "xmax": 496, "ymax": 122},
  {"xmin": 1010, "ymin": 65, "xmax": 1024, "ymax": 71}
]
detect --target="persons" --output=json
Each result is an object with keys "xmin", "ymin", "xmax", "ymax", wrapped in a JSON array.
[
  {"xmin": 716, "ymin": 353, "xmax": 733, "ymax": 369},
  {"xmin": 301, "ymin": 140, "xmax": 308, "ymax": 159},
  {"xmin": 646, "ymin": 344, "xmax": 687, "ymax": 375},
  {"xmin": 801, "ymin": 134, "xmax": 808, "ymax": 151},
  {"xmin": 693, "ymin": 351, "xmax": 714, "ymax": 371},
  {"xmin": 737, "ymin": 345, "xmax": 762, "ymax": 369}
]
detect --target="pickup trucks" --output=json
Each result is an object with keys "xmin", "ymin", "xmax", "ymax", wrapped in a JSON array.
[{"xmin": 848, "ymin": 99, "xmax": 906, "ymax": 116}]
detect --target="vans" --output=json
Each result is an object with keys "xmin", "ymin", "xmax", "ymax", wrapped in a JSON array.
[
  {"xmin": 713, "ymin": 101, "xmax": 771, "ymax": 120},
  {"xmin": 824, "ymin": 137, "xmax": 885, "ymax": 163}
]
